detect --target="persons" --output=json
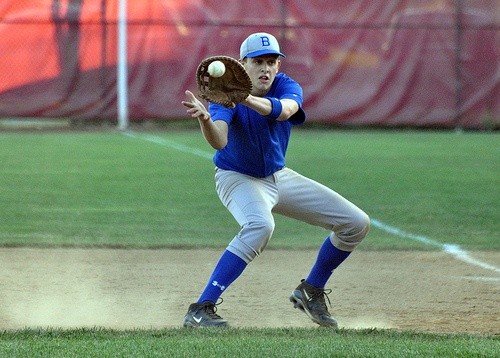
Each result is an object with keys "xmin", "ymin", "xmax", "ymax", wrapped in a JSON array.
[{"xmin": 181, "ymin": 32, "xmax": 371, "ymax": 329}]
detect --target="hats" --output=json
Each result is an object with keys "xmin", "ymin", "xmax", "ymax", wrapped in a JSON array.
[{"xmin": 240, "ymin": 32, "xmax": 286, "ymax": 60}]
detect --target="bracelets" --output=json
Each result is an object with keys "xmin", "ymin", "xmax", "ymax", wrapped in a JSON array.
[{"xmin": 260, "ymin": 96, "xmax": 283, "ymax": 121}]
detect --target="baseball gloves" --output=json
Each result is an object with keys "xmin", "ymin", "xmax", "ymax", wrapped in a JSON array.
[{"xmin": 196, "ymin": 55, "xmax": 253, "ymax": 107}]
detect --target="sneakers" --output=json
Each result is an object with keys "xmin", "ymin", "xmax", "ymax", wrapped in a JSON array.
[
  {"xmin": 289, "ymin": 279, "xmax": 338, "ymax": 327},
  {"xmin": 183, "ymin": 297, "xmax": 228, "ymax": 329}
]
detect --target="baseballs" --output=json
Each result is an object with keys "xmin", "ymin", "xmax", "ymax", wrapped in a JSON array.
[{"xmin": 208, "ymin": 60, "xmax": 225, "ymax": 77}]
